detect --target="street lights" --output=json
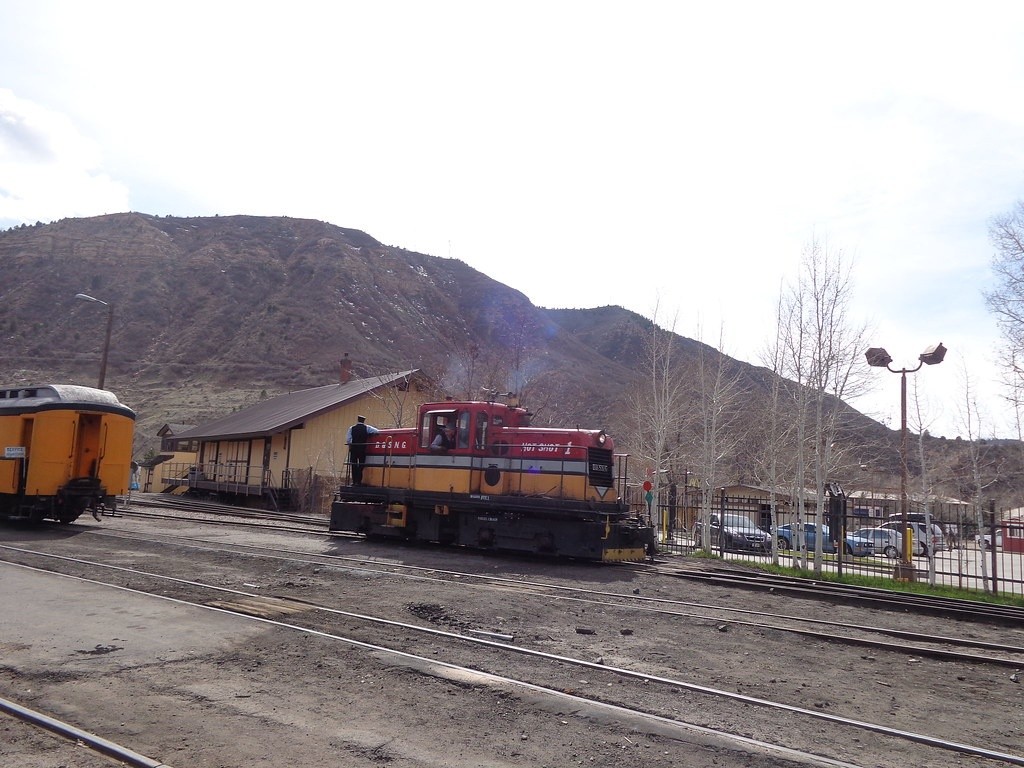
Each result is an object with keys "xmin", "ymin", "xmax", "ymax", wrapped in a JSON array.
[
  {"xmin": 863, "ymin": 340, "xmax": 949, "ymax": 583},
  {"xmin": 74, "ymin": 292, "xmax": 117, "ymax": 392},
  {"xmin": 861, "ymin": 465, "xmax": 884, "ymax": 526}
]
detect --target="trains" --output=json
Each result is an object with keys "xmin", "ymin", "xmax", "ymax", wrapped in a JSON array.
[
  {"xmin": 0, "ymin": 382, "xmax": 138, "ymax": 529},
  {"xmin": 327, "ymin": 385, "xmax": 657, "ymax": 564}
]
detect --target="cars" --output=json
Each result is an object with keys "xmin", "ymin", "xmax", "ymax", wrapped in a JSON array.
[
  {"xmin": 690, "ymin": 512, "xmax": 772, "ymax": 554},
  {"xmin": 777, "ymin": 522, "xmax": 875, "ymax": 558},
  {"xmin": 850, "ymin": 524, "xmax": 925, "ymax": 559}
]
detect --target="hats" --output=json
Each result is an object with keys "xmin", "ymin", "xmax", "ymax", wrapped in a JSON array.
[{"xmin": 358, "ymin": 415, "xmax": 366, "ymax": 421}]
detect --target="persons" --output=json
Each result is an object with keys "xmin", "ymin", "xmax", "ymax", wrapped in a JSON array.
[
  {"xmin": 430, "ymin": 423, "xmax": 467, "ymax": 451},
  {"xmin": 346, "ymin": 414, "xmax": 379, "ymax": 486}
]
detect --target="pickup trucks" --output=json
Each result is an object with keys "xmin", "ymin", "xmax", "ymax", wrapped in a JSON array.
[{"xmin": 974, "ymin": 529, "xmax": 1002, "ymax": 550}]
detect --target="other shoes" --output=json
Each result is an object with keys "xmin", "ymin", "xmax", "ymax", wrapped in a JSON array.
[{"xmin": 350, "ymin": 482, "xmax": 359, "ymax": 486}]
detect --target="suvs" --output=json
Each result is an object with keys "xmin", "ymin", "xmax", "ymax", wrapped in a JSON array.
[{"xmin": 877, "ymin": 511, "xmax": 949, "ymax": 556}]
